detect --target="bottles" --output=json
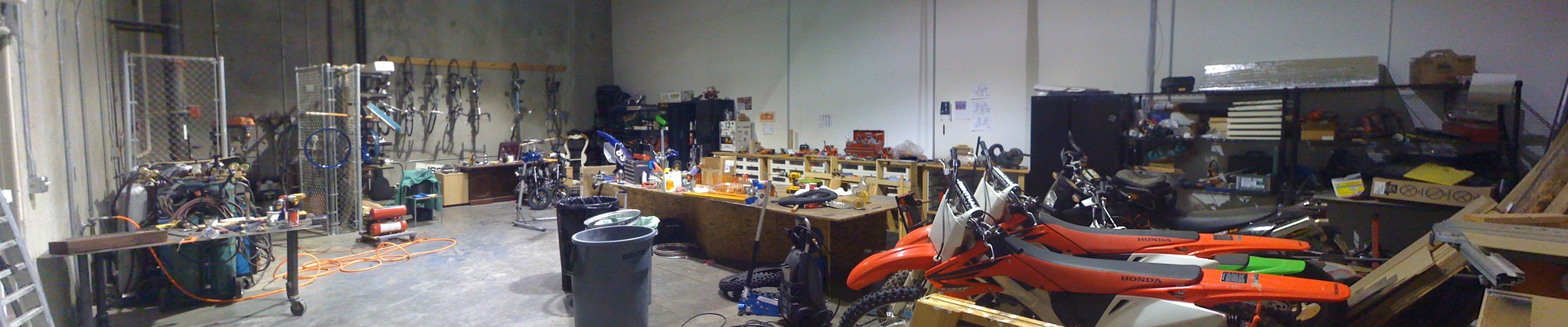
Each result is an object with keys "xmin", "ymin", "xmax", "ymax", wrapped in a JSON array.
[
  {"xmin": 619, "ymin": 168, "xmax": 624, "ymax": 183},
  {"xmin": 275, "ymin": 195, "xmax": 299, "ymax": 226},
  {"xmin": 725, "ymin": 109, "xmax": 729, "ymax": 121},
  {"xmin": 469, "ymin": 154, "xmax": 476, "ymax": 166},
  {"xmin": 752, "ymin": 181, "xmax": 764, "ymax": 206},
  {"xmin": 201, "ymin": 228, "xmax": 220, "ymax": 239},
  {"xmin": 642, "ymin": 169, "xmax": 647, "ymax": 184},
  {"xmin": 857, "ymin": 177, "xmax": 869, "ymax": 204}
]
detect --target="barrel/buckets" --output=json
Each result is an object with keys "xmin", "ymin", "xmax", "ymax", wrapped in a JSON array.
[{"xmin": 570, "ymin": 225, "xmax": 657, "ymax": 327}]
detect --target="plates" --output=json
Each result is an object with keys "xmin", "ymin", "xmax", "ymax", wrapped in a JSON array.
[{"xmin": 220, "ymin": 216, "xmax": 255, "ymax": 222}]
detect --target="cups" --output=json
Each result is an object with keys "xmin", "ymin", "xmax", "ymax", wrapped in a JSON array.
[
  {"xmin": 204, "ymin": 216, "xmax": 219, "ymax": 228},
  {"xmin": 648, "ymin": 178, "xmax": 656, "ymax": 185},
  {"xmin": 743, "ymin": 184, "xmax": 752, "ymax": 195},
  {"xmin": 266, "ymin": 212, "xmax": 280, "ymax": 225},
  {"xmin": 850, "ymin": 185, "xmax": 860, "ymax": 197},
  {"xmin": 809, "ymin": 183, "xmax": 817, "ymax": 190}
]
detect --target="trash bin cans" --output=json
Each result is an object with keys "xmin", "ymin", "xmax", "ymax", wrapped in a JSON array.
[
  {"xmin": 594, "ymin": 216, "xmax": 660, "ymax": 305},
  {"xmin": 556, "ymin": 195, "xmax": 619, "ymax": 293},
  {"xmin": 569, "ymin": 226, "xmax": 656, "ymax": 327}
]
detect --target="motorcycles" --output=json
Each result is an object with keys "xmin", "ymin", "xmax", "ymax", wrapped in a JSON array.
[
  {"xmin": 893, "ymin": 128, "xmax": 1335, "ymax": 327},
  {"xmin": 835, "ymin": 143, "xmax": 1349, "ymax": 327}
]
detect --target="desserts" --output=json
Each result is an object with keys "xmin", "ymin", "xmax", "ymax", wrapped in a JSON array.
[{"xmin": 299, "ymin": 210, "xmax": 307, "ymax": 220}]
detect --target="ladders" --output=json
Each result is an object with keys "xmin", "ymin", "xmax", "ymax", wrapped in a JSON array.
[{"xmin": 0, "ymin": 187, "xmax": 56, "ymax": 327}]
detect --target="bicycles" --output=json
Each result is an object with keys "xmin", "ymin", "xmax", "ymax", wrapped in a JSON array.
[
  {"xmin": 543, "ymin": 66, "xmax": 559, "ymax": 132},
  {"xmin": 376, "ymin": 55, "xmax": 491, "ymax": 137},
  {"xmin": 514, "ymin": 137, "xmax": 573, "ymax": 211},
  {"xmin": 506, "ymin": 62, "xmax": 536, "ymax": 139}
]
